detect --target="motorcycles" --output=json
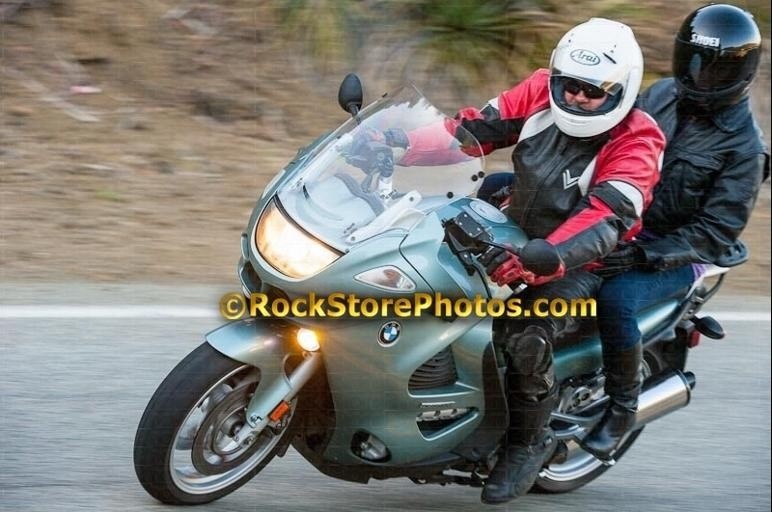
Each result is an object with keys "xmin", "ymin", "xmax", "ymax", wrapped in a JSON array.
[{"xmin": 134, "ymin": 75, "xmax": 748, "ymax": 505}]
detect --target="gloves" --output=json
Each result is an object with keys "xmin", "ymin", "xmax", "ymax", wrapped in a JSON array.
[
  {"xmin": 592, "ymin": 238, "xmax": 639, "ymax": 278},
  {"xmin": 482, "ymin": 238, "xmax": 566, "ymax": 287},
  {"xmin": 351, "ymin": 127, "xmax": 409, "ymax": 167}
]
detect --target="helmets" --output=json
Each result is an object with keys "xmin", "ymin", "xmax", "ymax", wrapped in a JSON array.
[
  {"xmin": 547, "ymin": 17, "xmax": 644, "ymax": 138},
  {"xmin": 673, "ymin": 4, "xmax": 761, "ymax": 113}
]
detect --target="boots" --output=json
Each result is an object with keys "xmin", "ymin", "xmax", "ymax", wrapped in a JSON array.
[
  {"xmin": 580, "ymin": 339, "xmax": 643, "ymax": 460},
  {"xmin": 480, "ymin": 390, "xmax": 557, "ymax": 504}
]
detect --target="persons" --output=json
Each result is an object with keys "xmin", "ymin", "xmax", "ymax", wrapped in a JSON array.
[
  {"xmin": 474, "ymin": 3, "xmax": 770, "ymax": 457},
  {"xmin": 344, "ymin": 17, "xmax": 664, "ymax": 507}
]
